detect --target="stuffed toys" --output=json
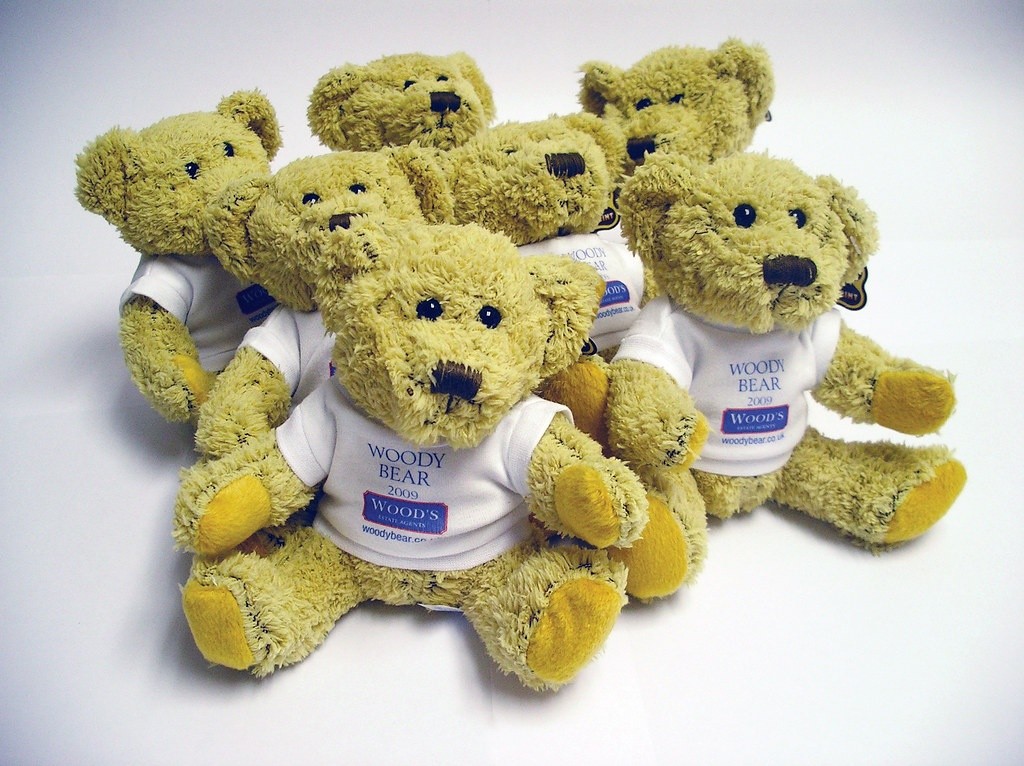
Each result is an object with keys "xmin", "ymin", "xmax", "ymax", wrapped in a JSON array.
[
  {"xmin": 69, "ymin": 33, "xmax": 771, "ymax": 695},
  {"xmin": 604, "ymin": 147, "xmax": 967, "ymax": 601}
]
